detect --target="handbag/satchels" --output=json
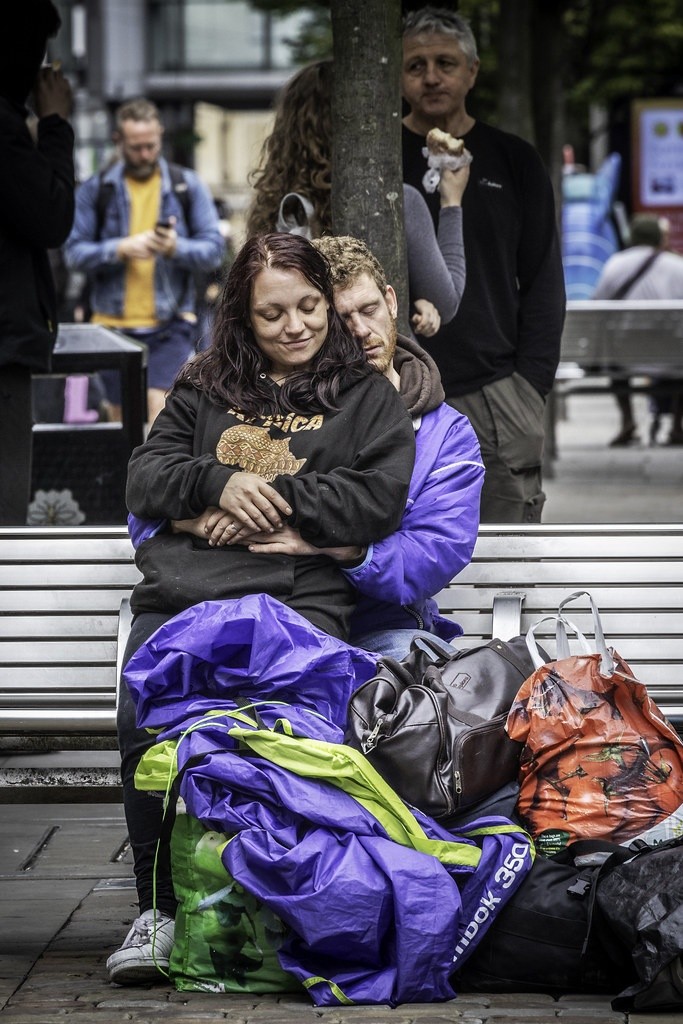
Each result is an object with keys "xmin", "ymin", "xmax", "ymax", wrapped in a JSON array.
[{"xmin": 346, "ymin": 589, "xmax": 683, "ymax": 1015}]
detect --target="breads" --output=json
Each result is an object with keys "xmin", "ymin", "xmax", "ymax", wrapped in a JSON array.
[{"xmin": 426, "ymin": 127, "xmax": 464, "ymax": 156}]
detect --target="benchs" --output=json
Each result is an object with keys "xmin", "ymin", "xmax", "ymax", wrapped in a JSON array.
[
  {"xmin": 545, "ymin": 300, "xmax": 683, "ymax": 480},
  {"xmin": 0, "ymin": 522, "xmax": 683, "ymax": 806}
]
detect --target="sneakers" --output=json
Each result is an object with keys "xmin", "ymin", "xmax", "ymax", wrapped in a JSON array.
[{"xmin": 105, "ymin": 906, "xmax": 177, "ymax": 985}]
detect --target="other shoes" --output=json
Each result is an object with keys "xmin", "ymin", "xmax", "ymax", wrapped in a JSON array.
[{"xmin": 609, "ymin": 426, "xmax": 640, "ymax": 447}]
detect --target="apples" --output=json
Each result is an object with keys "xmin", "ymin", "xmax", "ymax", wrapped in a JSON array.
[{"xmin": 194, "ymin": 830, "xmax": 249, "ymax": 956}]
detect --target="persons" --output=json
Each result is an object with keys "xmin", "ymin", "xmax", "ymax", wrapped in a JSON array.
[
  {"xmin": 402, "ymin": 10, "xmax": 568, "ymax": 524},
  {"xmin": 559, "ymin": 144, "xmax": 630, "ymax": 373},
  {"xmin": 0, "ymin": 0, "xmax": 82, "ymax": 526},
  {"xmin": 242, "ymin": 44, "xmax": 470, "ymax": 359},
  {"xmin": 62, "ymin": 96, "xmax": 228, "ymax": 452},
  {"xmin": 102, "ymin": 224, "xmax": 487, "ymax": 989},
  {"xmin": 590, "ymin": 214, "xmax": 682, "ymax": 447}
]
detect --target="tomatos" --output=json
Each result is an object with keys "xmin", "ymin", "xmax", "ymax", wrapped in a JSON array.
[{"xmin": 520, "ymin": 719, "xmax": 683, "ymax": 855}]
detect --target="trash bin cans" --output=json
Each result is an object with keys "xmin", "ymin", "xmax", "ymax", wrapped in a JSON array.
[{"xmin": 31, "ymin": 323, "xmax": 149, "ymax": 521}]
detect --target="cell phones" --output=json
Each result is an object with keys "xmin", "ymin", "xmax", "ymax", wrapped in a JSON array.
[{"xmin": 156, "ymin": 221, "xmax": 170, "ymax": 237}]
